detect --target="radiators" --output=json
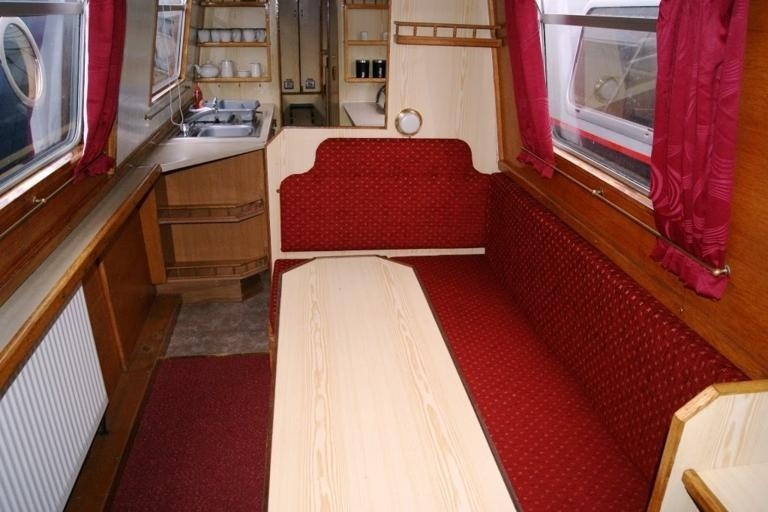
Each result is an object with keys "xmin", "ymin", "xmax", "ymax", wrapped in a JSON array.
[{"xmin": 0, "ymin": 279, "xmax": 111, "ymax": 512}]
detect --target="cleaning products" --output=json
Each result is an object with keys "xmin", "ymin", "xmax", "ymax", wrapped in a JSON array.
[{"xmin": 192, "ymin": 82, "xmax": 203, "ymax": 109}]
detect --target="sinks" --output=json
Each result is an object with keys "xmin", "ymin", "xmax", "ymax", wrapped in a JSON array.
[
  {"xmin": 198, "ymin": 126, "xmax": 254, "ymax": 140},
  {"xmin": 204, "ymin": 101, "xmax": 257, "ymax": 109},
  {"xmin": 194, "ymin": 110, "xmax": 255, "ymax": 123}
]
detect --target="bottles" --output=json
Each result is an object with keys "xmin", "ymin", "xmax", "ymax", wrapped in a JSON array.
[
  {"xmin": 220, "ymin": 58, "xmax": 234, "ymax": 79},
  {"xmin": 250, "ymin": 62, "xmax": 262, "ymax": 77},
  {"xmin": 193, "ymin": 86, "xmax": 203, "ymax": 108}
]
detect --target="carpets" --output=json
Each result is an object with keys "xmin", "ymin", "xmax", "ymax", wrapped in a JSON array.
[{"xmin": 98, "ymin": 353, "xmax": 269, "ymax": 512}]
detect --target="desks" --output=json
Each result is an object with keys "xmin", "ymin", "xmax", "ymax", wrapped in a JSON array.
[{"xmin": 268, "ymin": 241, "xmax": 523, "ymax": 512}]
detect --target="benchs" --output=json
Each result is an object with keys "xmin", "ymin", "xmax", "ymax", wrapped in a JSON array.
[{"xmin": 259, "ymin": 126, "xmax": 768, "ymax": 512}]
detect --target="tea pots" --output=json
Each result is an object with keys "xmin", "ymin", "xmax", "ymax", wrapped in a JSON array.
[{"xmin": 195, "ymin": 62, "xmax": 217, "ymax": 79}]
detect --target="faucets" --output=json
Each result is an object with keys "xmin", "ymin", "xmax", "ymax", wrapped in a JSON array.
[
  {"xmin": 212, "ymin": 97, "xmax": 219, "ymax": 112},
  {"xmin": 180, "ymin": 133, "xmax": 192, "ymax": 137},
  {"xmin": 180, "ymin": 121, "xmax": 199, "ymax": 132}
]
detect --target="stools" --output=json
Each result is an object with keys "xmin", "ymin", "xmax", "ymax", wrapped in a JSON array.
[{"xmin": 289, "ymin": 103, "xmax": 315, "ymax": 125}]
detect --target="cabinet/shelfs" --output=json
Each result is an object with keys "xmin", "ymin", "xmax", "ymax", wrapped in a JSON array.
[
  {"xmin": 341, "ymin": 0, "xmax": 390, "ymax": 84},
  {"xmin": 153, "ymin": 138, "xmax": 268, "ymax": 308},
  {"xmin": 194, "ymin": 0, "xmax": 274, "ymax": 90},
  {"xmin": 278, "ymin": 1, "xmax": 322, "ymax": 95}
]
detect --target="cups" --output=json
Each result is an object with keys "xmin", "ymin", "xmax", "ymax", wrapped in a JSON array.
[
  {"xmin": 197, "ymin": 28, "xmax": 267, "ymax": 44},
  {"xmin": 372, "ymin": 60, "xmax": 385, "ymax": 77},
  {"xmin": 382, "ymin": 31, "xmax": 388, "ymax": 40},
  {"xmin": 361, "ymin": 31, "xmax": 368, "ymax": 40},
  {"xmin": 239, "ymin": 71, "xmax": 250, "ymax": 78},
  {"xmin": 355, "ymin": 59, "xmax": 370, "ymax": 78}
]
